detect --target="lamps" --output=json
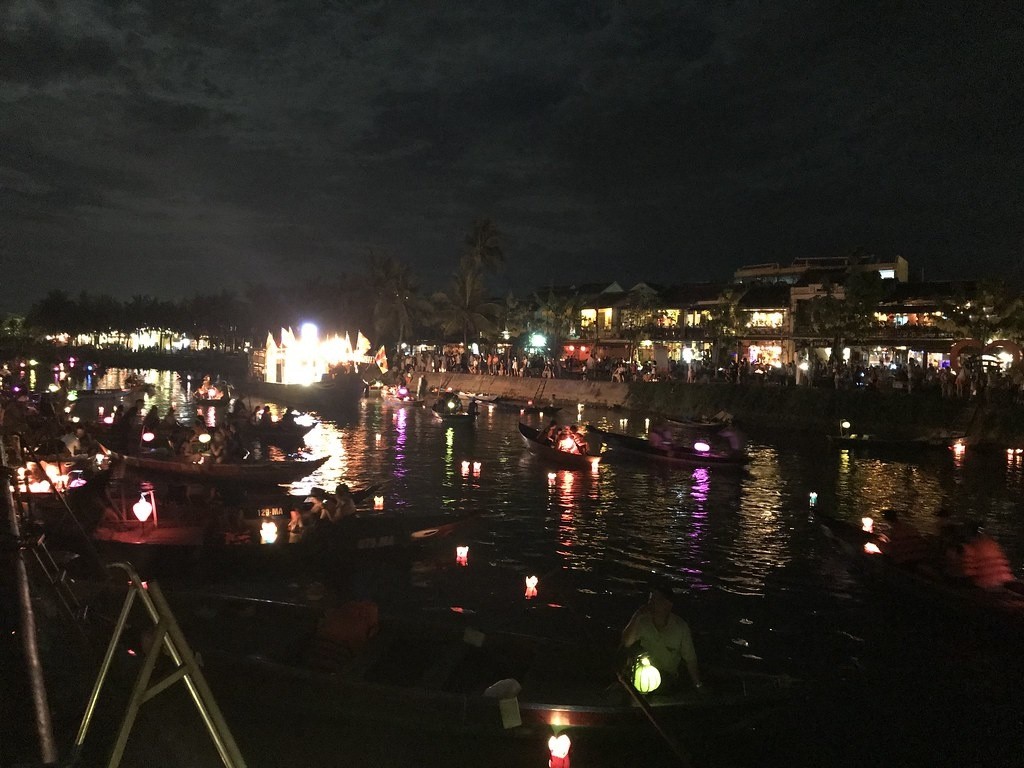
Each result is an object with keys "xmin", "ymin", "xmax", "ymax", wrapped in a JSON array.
[
  {"xmin": 456, "ymin": 542, "xmax": 469, "ymax": 567},
  {"xmin": 525, "ymin": 572, "xmax": 538, "ymax": 599},
  {"xmin": 133, "ymin": 496, "xmax": 153, "ymax": 521},
  {"xmin": 630, "ymin": 639, "xmax": 661, "ymax": 692},
  {"xmin": 374, "ymin": 491, "xmax": 384, "ymax": 510},
  {"xmin": 260, "ymin": 516, "xmax": 278, "ymax": 544},
  {"xmin": 548, "ymin": 725, "xmax": 571, "ymax": 768}
]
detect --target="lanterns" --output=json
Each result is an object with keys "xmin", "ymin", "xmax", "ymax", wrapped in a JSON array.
[{"xmin": 632, "ymin": 658, "xmax": 661, "ymax": 695}]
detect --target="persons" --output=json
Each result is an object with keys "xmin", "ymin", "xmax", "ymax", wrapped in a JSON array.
[
  {"xmin": 0, "ymin": 354, "xmax": 357, "ymax": 546},
  {"xmin": 389, "ymin": 340, "xmax": 1024, "ymax": 400},
  {"xmin": 546, "ymin": 394, "xmax": 557, "ymax": 409},
  {"xmin": 536, "ymin": 421, "xmax": 740, "ymax": 459},
  {"xmin": 619, "ymin": 581, "xmax": 705, "ymax": 697},
  {"xmin": 430, "ymin": 393, "xmax": 479, "ymax": 416}
]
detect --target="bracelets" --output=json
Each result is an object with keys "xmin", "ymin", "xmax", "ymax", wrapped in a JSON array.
[{"xmin": 694, "ymin": 678, "xmax": 705, "ymax": 689}]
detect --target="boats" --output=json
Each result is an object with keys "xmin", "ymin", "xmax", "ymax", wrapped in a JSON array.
[
  {"xmin": 223, "ymin": 412, "xmax": 317, "ymax": 442},
  {"xmin": 431, "ymin": 393, "xmax": 476, "ymax": 424},
  {"xmin": 518, "ymin": 422, "xmax": 602, "ymax": 468},
  {"xmin": 495, "ymin": 398, "xmax": 563, "ymax": 415},
  {"xmin": 94, "ymin": 494, "xmax": 487, "ymax": 578},
  {"xmin": 585, "ymin": 424, "xmax": 756, "ymax": 472},
  {"xmin": 44, "ymin": 577, "xmax": 805, "ymax": 768},
  {"xmin": 828, "ymin": 434, "xmax": 954, "ymax": 455},
  {"xmin": 240, "ymin": 368, "xmax": 368, "ymax": 406},
  {"xmin": 93, "ymin": 436, "xmax": 331, "ymax": 485},
  {"xmin": 807, "ymin": 505, "xmax": 1024, "ymax": 626}
]
